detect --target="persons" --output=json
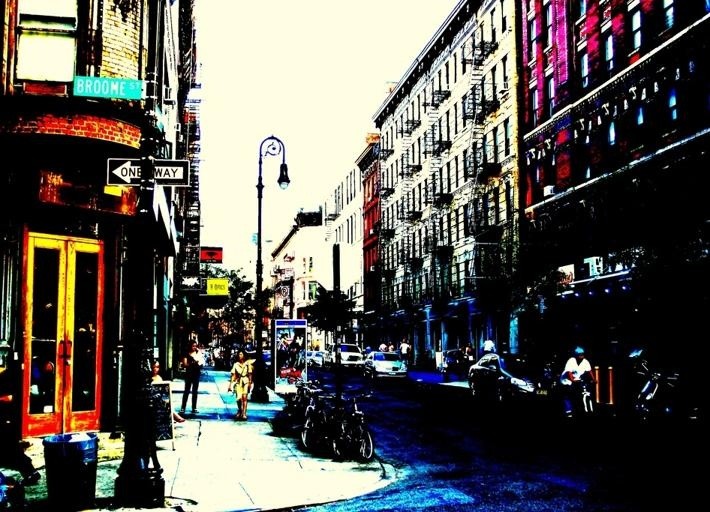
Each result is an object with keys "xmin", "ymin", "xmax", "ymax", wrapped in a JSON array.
[
  {"xmin": 150, "ymin": 361, "xmax": 185, "ymax": 422},
  {"xmin": 483, "ymin": 337, "xmax": 497, "ymax": 355},
  {"xmin": 465, "ymin": 343, "xmax": 474, "ymax": 357},
  {"xmin": 180, "ymin": 341, "xmax": 203, "ymax": 413},
  {"xmin": 228, "ymin": 350, "xmax": 253, "ymax": 419},
  {"xmin": 379, "ymin": 339, "xmax": 409, "ymax": 362},
  {"xmin": 560, "ymin": 347, "xmax": 594, "ymax": 417},
  {"xmin": 203, "ymin": 344, "xmax": 222, "ymax": 366}
]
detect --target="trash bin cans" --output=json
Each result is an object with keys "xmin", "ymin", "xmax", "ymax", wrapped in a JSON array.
[{"xmin": 42, "ymin": 432, "xmax": 99, "ymax": 512}]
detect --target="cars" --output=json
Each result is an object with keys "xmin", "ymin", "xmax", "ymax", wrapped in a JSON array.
[
  {"xmin": 466, "ymin": 352, "xmax": 558, "ymax": 405},
  {"xmin": 306, "ymin": 344, "xmax": 408, "ymax": 381}
]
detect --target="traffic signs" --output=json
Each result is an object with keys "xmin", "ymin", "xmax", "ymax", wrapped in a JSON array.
[{"xmin": 104, "ymin": 158, "xmax": 190, "ymax": 187}]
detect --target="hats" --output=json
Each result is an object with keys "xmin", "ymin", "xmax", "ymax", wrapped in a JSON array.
[{"xmin": 575, "ymin": 347, "xmax": 584, "ymax": 354}]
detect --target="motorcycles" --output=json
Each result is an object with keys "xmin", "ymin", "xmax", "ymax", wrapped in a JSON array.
[{"xmin": 634, "ymin": 359, "xmax": 704, "ymax": 426}]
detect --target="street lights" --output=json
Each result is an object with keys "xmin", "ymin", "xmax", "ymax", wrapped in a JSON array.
[{"xmin": 251, "ymin": 132, "xmax": 290, "ymax": 404}]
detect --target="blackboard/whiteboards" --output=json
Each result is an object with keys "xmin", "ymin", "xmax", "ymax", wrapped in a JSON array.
[{"xmin": 153, "ymin": 383, "xmax": 174, "ymax": 441}]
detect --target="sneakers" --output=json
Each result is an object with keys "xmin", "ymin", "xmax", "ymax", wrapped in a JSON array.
[
  {"xmin": 180, "ymin": 409, "xmax": 198, "ymax": 413},
  {"xmin": 235, "ymin": 409, "xmax": 247, "ymax": 420}
]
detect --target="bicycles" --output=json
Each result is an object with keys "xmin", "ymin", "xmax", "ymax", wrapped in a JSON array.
[
  {"xmin": 273, "ymin": 379, "xmax": 376, "ymax": 463},
  {"xmin": 558, "ymin": 376, "xmax": 594, "ymax": 423}
]
